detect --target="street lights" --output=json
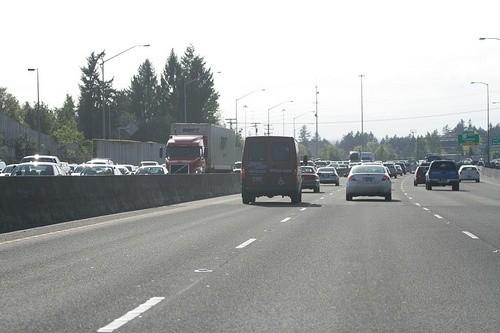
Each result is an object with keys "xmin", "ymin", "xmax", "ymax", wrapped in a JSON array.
[
  {"xmin": 268, "ymin": 100, "xmax": 294, "ymax": 136},
  {"xmin": 236, "ymin": 88, "xmax": 265, "ymax": 137},
  {"xmin": 99, "ymin": 45, "xmax": 151, "ymax": 139},
  {"xmin": 294, "ymin": 110, "xmax": 315, "ymax": 138},
  {"xmin": 28, "ymin": 68, "xmax": 42, "ymax": 154},
  {"xmin": 183, "ymin": 71, "xmax": 221, "ymax": 123},
  {"xmin": 471, "ymin": 81, "xmax": 490, "ymax": 165}
]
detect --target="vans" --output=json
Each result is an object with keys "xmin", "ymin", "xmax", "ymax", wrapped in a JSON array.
[
  {"xmin": 241, "ymin": 136, "xmax": 302, "ymax": 204},
  {"xmin": 233, "ymin": 162, "xmax": 241, "ymax": 172}
]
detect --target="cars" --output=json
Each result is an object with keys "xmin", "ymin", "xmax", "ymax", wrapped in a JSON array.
[
  {"xmin": 414, "ymin": 156, "xmax": 500, "ymax": 190},
  {"xmin": 346, "ymin": 165, "xmax": 391, "ymax": 201},
  {"xmin": 299, "ymin": 160, "xmax": 418, "ymax": 193},
  {"xmin": 0, "ymin": 155, "xmax": 168, "ymax": 176}
]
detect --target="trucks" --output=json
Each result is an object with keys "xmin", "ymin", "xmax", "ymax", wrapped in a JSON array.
[
  {"xmin": 160, "ymin": 123, "xmax": 235, "ymax": 173},
  {"xmin": 348, "ymin": 151, "xmax": 375, "ymax": 162}
]
